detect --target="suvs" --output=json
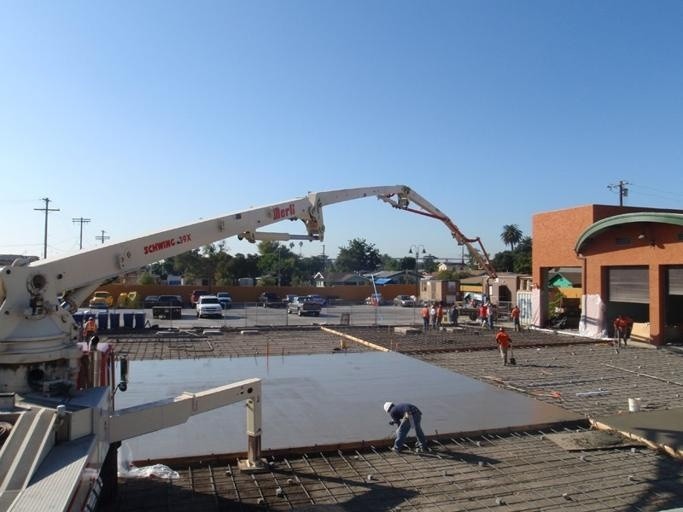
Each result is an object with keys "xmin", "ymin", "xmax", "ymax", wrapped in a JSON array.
[
  {"xmin": 217, "ymin": 291, "xmax": 233, "ymax": 309},
  {"xmin": 194, "ymin": 295, "xmax": 224, "ymax": 319},
  {"xmin": 188, "ymin": 290, "xmax": 208, "ymax": 308}
]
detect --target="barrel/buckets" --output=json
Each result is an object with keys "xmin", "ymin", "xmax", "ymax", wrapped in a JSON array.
[{"xmin": 628, "ymin": 397, "xmax": 642, "ymax": 412}]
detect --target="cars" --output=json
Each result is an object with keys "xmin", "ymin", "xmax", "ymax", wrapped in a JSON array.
[
  {"xmin": 152, "ymin": 295, "xmax": 185, "ymax": 319},
  {"xmin": 365, "ymin": 293, "xmax": 385, "ymax": 304},
  {"xmin": 280, "ymin": 294, "xmax": 326, "ymax": 315},
  {"xmin": 257, "ymin": 291, "xmax": 282, "ymax": 307},
  {"xmin": 393, "ymin": 285, "xmax": 489, "ymax": 322},
  {"xmin": 144, "ymin": 295, "xmax": 158, "ymax": 308},
  {"xmin": 88, "ymin": 291, "xmax": 114, "ymax": 309}
]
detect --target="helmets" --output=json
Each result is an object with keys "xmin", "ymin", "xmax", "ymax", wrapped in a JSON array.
[{"xmin": 384, "ymin": 402, "xmax": 393, "ymax": 414}]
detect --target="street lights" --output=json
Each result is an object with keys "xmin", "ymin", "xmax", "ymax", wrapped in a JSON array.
[
  {"xmin": 275, "ymin": 244, "xmax": 283, "ymax": 288},
  {"xmin": 407, "ymin": 240, "xmax": 427, "ymax": 299}
]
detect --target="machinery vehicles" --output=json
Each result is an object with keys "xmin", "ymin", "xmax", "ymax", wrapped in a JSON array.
[{"xmin": 0, "ymin": 182, "xmax": 511, "ymax": 511}]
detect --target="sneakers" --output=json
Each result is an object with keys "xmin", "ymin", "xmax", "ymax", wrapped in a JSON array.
[{"xmin": 392, "ymin": 447, "xmax": 400, "ymax": 452}]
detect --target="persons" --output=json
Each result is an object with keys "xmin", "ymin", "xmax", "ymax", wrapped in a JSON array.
[
  {"xmin": 420, "ymin": 298, "xmax": 494, "ymax": 331},
  {"xmin": 495, "ymin": 326, "xmax": 512, "ymax": 366},
  {"xmin": 510, "ymin": 304, "xmax": 521, "ymax": 332},
  {"xmin": 383, "ymin": 401, "xmax": 429, "ymax": 452},
  {"xmin": 614, "ymin": 312, "xmax": 633, "ymax": 347},
  {"xmin": 83, "ymin": 316, "xmax": 97, "ymax": 343}
]
